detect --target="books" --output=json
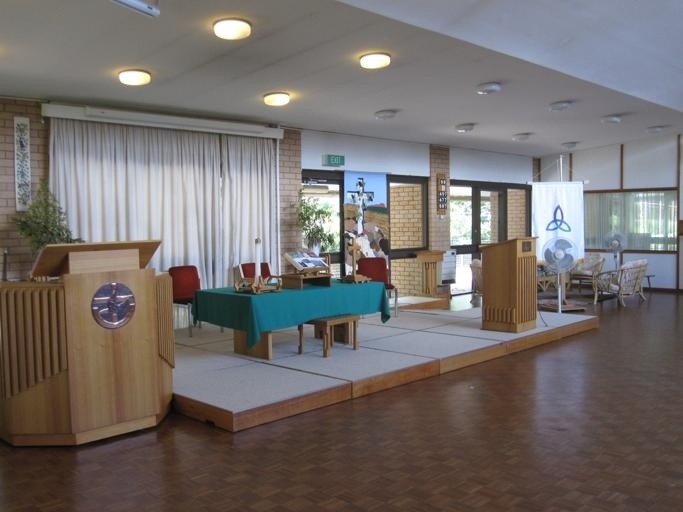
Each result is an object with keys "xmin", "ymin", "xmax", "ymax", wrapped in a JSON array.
[{"xmin": 284, "ymin": 249, "xmax": 328, "ymax": 271}]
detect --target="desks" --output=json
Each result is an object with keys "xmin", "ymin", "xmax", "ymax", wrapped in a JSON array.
[{"xmin": 187, "ymin": 277, "xmax": 392, "ymax": 363}]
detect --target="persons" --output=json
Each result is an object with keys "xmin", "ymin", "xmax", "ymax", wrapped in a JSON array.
[{"xmin": 378, "ymin": 239, "xmax": 389, "ymax": 270}]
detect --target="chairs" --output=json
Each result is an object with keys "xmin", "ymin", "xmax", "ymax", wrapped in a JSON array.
[
  {"xmin": 162, "ymin": 265, "xmax": 224, "ymax": 338},
  {"xmin": 357, "ymin": 255, "xmax": 399, "ymax": 319},
  {"xmin": 236, "ymin": 261, "xmax": 272, "ymax": 284},
  {"xmin": 468, "ymin": 252, "xmax": 655, "ymax": 311}
]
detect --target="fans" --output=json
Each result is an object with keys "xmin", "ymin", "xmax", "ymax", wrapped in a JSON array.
[
  {"xmin": 540, "ymin": 235, "xmax": 579, "ymax": 313},
  {"xmin": 604, "ymin": 229, "xmax": 629, "ymax": 296}
]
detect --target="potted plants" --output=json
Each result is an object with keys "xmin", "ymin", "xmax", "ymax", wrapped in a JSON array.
[{"xmin": 285, "ymin": 189, "xmax": 342, "ymax": 255}]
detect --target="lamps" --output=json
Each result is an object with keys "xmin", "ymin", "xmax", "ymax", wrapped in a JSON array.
[
  {"xmin": 563, "ymin": 141, "xmax": 580, "ymax": 149},
  {"xmin": 213, "ymin": 19, "xmax": 253, "ymax": 40},
  {"xmin": 601, "ymin": 114, "xmax": 622, "ymax": 124},
  {"xmin": 455, "ymin": 121, "xmax": 479, "ymax": 133},
  {"xmin": 548, "ymin": 100, "xmax": 572, "ymax": 112},
  {"xmin": 375, "ymin": 110, "xmax": 397, "ymax": 118},
  {"xmin": 477, "ymin": 82, "xmax": 503, "ymax": 95},
  {"xmin": 264, "ymin": 93, "xmax": 292, "ymax": 107},
  {"xmin": 359, "ymin": 53, "xmax": 391, "ymax": 70},
  {"xmin": 647, "ymin": 125, "xmax": 663, "ymax": 134},
  {"xmin": 119, "ymin": 70, "xmax": 151, "ymax": 87},
  {"xmin": 514, "ymin": 132, "xmax": 531, "ymax": 142}
]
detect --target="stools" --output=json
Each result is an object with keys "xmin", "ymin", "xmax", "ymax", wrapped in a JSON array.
[{"xmin": 297, "ymin": 311, "xmax": 359, "ymax": 357}]
